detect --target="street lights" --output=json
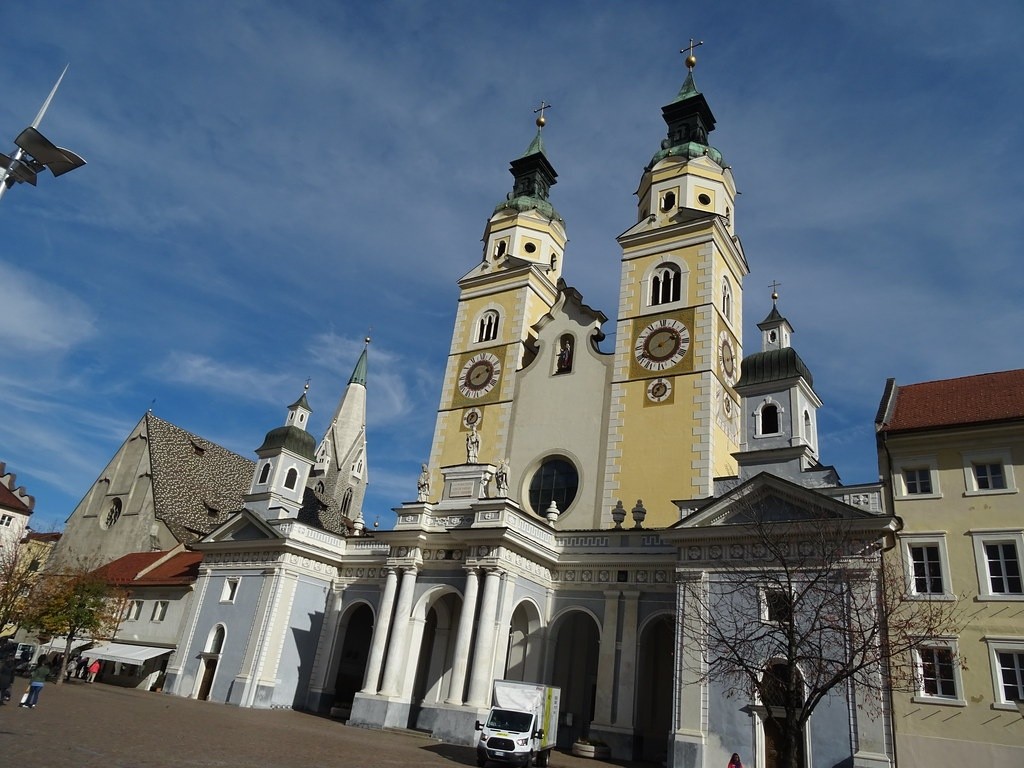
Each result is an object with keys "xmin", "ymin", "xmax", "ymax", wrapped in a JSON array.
[{"xmin": 0, "ymin": 63, "xmax": 88, "ymax": 198}]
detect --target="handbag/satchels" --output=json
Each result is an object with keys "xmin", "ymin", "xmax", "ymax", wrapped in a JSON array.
[
  {"xmin": 23, "ymin": 684, "xmax": 30, "ymax": 693},
  {"xmin": 87, "ymin": 672, "xmax": 90, "ymax": 678}
]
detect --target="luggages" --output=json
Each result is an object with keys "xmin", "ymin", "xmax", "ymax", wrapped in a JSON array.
[{"xmin": 17, "ymin": 692, "xmax": 29, "ymax": 707}]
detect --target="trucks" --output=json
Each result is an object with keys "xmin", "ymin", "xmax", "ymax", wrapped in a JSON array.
[{"xmin": 475, "ymin": 680, "xmax": 563, "ymax": 768}]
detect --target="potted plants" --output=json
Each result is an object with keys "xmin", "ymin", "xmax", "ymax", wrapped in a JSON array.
[{"xmin": 571, "ymin": 737, "xmax": 610, "ymax": 759}]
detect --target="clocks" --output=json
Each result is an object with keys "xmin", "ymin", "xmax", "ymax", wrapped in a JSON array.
[
  {"xmin": 719, "ymin": 330, "xmax": 737, "ymax": 388},
  {"xmin": 634, "ymin": 318, "xmax": 690, "ymax": 372},
  {"xmin": 458, "ymin": 352, "xmax": 502, "ymax": 399},
  {"xmin": 462, "ymin": 407, "xmax": 482, "ymax": 429},
  {"xmin": 723, "ymin": 387, "xmax": 732, "ymax": 423},
  {"xmin": 646, "ymin": 378, "xmax": 671, "ymax": 403}
]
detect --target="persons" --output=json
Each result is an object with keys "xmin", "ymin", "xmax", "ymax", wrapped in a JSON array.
[
  {"xmin": 0, "ymin": 652, "xmax": 51, "ymax": 709},
  {"xmin": 21, "ymin": 646, "xmax": 102, "ymax": 684},
  {"xmin": 416, "ymin": 460, "xmax": 432, "ymax": 504},
  {"xmin": 495, "ymin": 460, "xmax": 512, "ymax": 498},
  {"xmin": 556, "ymin": 339, "xmax": 572, "ymax": 371},
  {"xmin": 464, "ymin": 424, "xmax": 482, "ymax": 464},
  {"xmin": 728, "ymin": 751, "xmax": 743, "ymax": 768}
]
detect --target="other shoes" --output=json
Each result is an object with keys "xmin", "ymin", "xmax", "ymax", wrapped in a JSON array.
[
  {"xmin": 31, "ymin": 705, "xmax": 35, "ymax": 710},
  {"xmin": 89, "ymin": 682, "xmax": 93, "ymax": 684},
  {"xmin": 23, "ymin": 705, "xmax": 28, "ymax": 708},
  {"xmin": 85, "ymin": 680, "xmax": 90, "ymax": 683}
]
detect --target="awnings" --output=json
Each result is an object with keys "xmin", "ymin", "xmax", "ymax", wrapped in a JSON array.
[
  {"xmin": 45, "ymin": 636, "xmax": 90, "ymax": 654},
  {"xmin": 82, "ymin": 641, "xmax": 176, "ymax": 667}
]
